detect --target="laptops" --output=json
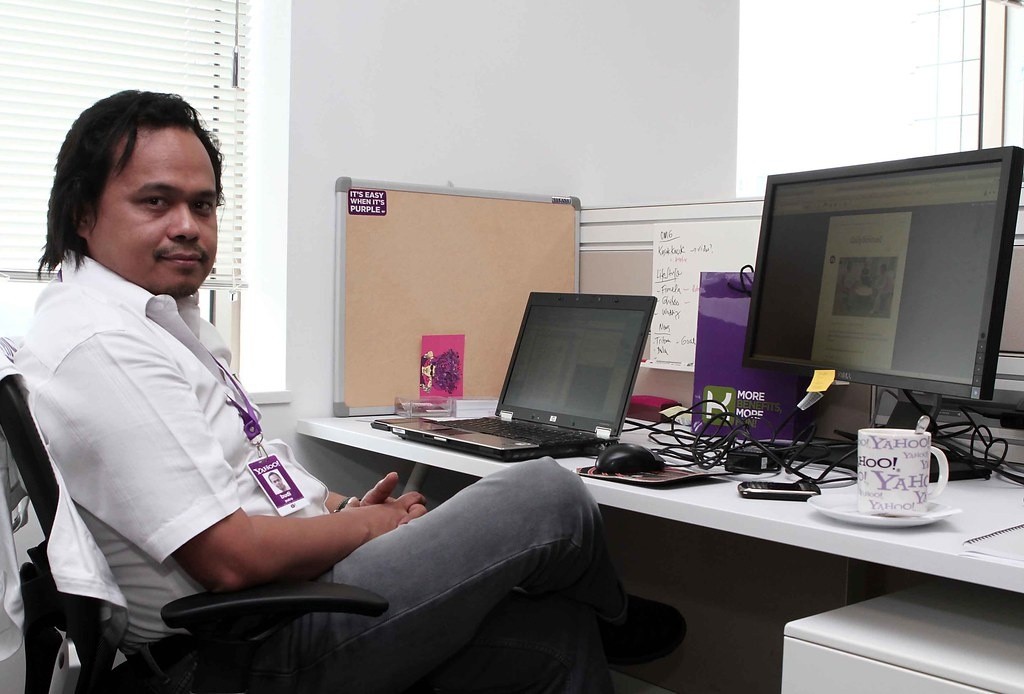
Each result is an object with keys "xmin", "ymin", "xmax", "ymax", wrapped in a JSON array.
[{"xmin": 372, "ymin": 292, "xmax": 658, "ymax": 461}]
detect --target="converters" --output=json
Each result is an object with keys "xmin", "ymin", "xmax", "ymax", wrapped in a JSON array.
[{"xmin": 724, "ymin": 445, "xmax": 781, "ymax": 474}]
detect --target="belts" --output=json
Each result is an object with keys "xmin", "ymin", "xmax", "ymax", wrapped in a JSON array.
[{"xmin": 126, "ymin": 633, "xmax": 190, "ymax": 678}]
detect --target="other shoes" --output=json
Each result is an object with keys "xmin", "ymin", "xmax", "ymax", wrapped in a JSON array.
[{"xmin": 595, "ymin": 593, "xmax": 687, "ymax": 665}]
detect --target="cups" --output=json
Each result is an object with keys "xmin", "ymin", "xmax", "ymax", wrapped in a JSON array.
[{"xmin": 858, "ymin": 428, "xmax": 949, "ymax": 516}]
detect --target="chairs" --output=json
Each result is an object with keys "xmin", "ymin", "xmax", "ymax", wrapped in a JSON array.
[{"xmin": 0, "ymin": 371, "xmax": 389, "ymax": 694}]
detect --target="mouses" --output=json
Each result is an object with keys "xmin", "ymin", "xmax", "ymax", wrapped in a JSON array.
[{"xmin": 595, "ymin": 442, "xmax": 667, "ymax": 473}]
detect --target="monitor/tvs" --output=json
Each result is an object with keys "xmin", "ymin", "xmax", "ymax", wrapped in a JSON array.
[{"xmin": 746, "ymin": 146, "xmax": 1024, "ymax": 481}]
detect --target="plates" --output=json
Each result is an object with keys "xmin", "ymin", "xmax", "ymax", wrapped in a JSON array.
[{"xmin": 807, "ymin": 492, "xmax": 961, "ymax": 527}]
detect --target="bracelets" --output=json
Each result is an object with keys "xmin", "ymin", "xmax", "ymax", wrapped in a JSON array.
[{"xmin": 335, "ymin": 498, "xmax": 362, "ymax": 513}]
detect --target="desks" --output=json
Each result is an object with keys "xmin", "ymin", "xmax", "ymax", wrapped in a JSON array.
[{"xmin": 298, "ymin": 418, "xmax": 1024, "ymax": 694}]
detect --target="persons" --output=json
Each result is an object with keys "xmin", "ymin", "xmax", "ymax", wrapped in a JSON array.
[
  {"xmin": 19, "ymin": 90, "xmax": 689, "ymax": 694},
  {"xmin": 837, "ymin": 262, "xmax": 895, "ymax": 317}
]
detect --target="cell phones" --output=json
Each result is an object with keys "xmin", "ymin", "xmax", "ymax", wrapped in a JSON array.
[{"xmin": 738, "ymin": 481, "xmax": 821, "ymax": 502}]
